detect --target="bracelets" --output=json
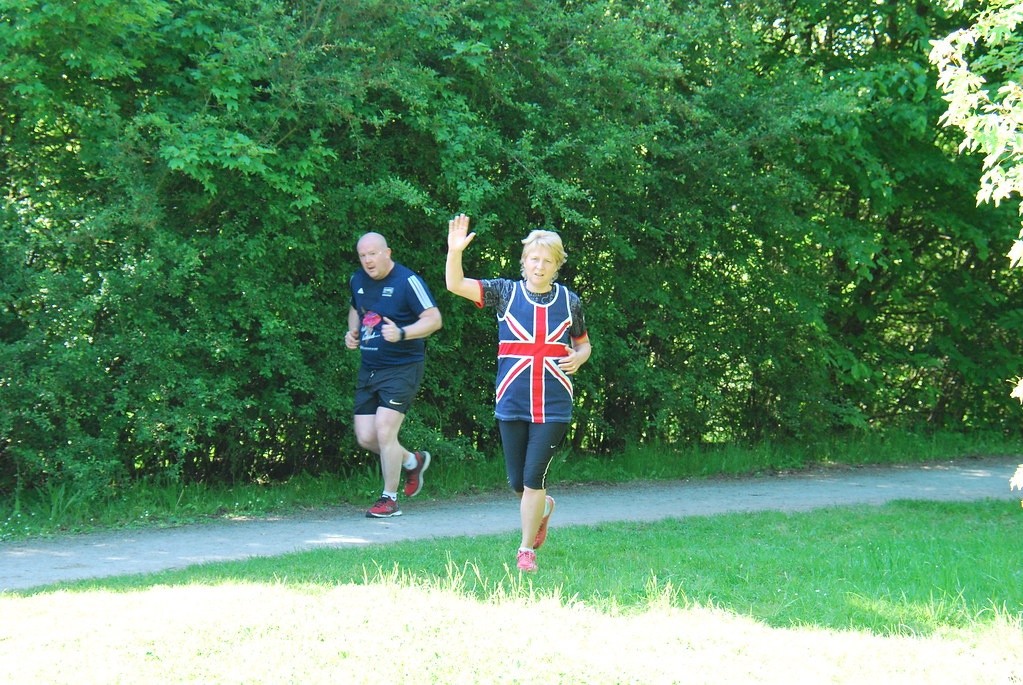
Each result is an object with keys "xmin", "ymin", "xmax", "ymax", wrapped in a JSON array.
[{"xmin": 400, "ymin": 327, "xmax": 405, "ymax": 342}]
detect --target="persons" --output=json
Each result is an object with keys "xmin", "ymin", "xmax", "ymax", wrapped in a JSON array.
[
  {"xmin": 444, "ymin": 213, "xmax": 592, "ymax": 573},
  {"xmin": 344, "ymin": 232, "xmax": 443, "ymax": 518}
]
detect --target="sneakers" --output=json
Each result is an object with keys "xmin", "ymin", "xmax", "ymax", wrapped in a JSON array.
[
  {"xmin": 532, "ymin": 494, "xmax": 555, "ymax": 548},
  {"xmin": 402, "ymin": 451, "xmax": 431, "ymax": 497},
  {"xmin": 516, "ymin": 547, "xmax": 538, "ymax": 573},
  {"xmin": 366, "ymin": 495, "xmax": 402, "ymax": 518}
]
